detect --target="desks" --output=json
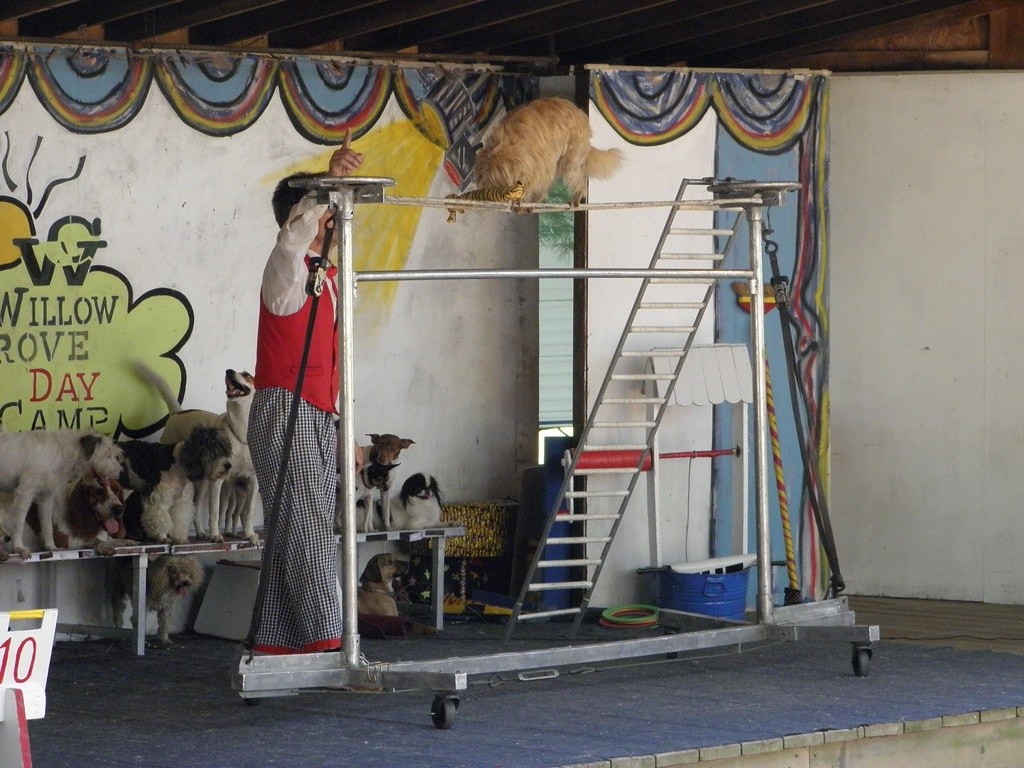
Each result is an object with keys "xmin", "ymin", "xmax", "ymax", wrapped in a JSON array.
[{"xmin": 0, "ymin": 523, "xmax": 470, "ymax": 654}]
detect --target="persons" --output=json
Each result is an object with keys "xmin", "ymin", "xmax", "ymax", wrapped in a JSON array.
[{"xmin": 245, "ymin": 121, "xmax": 370, "ymax": 654}]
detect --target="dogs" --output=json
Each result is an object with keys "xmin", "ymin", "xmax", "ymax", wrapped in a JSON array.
[
  {"xmin": 106, "ymin": 553, "xmax": 204, "ymax": 647},
  {"xmin": 111, "ymin": 369, "xmax": 260, "ymax": 545},
  {"xmin": 356, "ymin": 553, "xmax": 438, "ymax": 636},
  {"xmin": 329, "ymin": 425, "xmax": 418, "ymax": 533},
  {"xmin": 0, "ymin": 429, "xmax": 136, "ymax": 559},
  {"xmin": 355, "ymin": 471, "xmax": 462, "ymax": 530},
  {"xmin": 474, "ymin": 94, "xmax": 626, "ymax": 206}
]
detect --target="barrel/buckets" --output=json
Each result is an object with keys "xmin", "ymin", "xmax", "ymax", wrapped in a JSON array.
[{"xmin": 649, "ymin": 567, "xmax": 750, "ymax": 621}]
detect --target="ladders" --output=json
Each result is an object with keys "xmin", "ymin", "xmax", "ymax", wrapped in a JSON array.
[{"xmin": 506, "ymin": 180, "xmax": 746, "ymax": 637}]
